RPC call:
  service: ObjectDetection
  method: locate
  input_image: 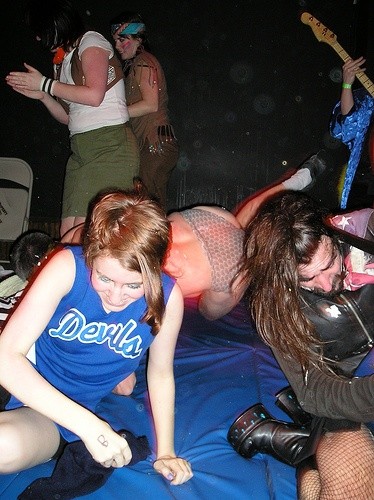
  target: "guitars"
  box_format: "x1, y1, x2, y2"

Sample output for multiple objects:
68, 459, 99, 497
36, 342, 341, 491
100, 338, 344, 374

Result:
301, 12, 374, 99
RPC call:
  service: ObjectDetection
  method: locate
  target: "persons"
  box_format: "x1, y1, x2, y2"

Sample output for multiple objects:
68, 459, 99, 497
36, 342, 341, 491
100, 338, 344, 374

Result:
228, 188, 374, 499
330, 57, 374, 215
5, 0, 142, 240
0, 192, 194, 485
9, 148, 330, 321
111, 12, 180, 210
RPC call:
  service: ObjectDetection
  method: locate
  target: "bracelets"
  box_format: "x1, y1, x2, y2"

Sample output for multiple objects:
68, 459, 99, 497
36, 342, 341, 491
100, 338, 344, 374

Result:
40, 76, 59, 96
342, 83, 351, 89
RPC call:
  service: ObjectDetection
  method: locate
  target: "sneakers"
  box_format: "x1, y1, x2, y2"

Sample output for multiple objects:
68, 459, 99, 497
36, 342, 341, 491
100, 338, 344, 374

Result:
299, 148, 333, 193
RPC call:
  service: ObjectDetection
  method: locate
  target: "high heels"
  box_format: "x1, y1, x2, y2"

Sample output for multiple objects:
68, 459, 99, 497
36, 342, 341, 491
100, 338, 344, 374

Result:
226, 383, 322, 479
